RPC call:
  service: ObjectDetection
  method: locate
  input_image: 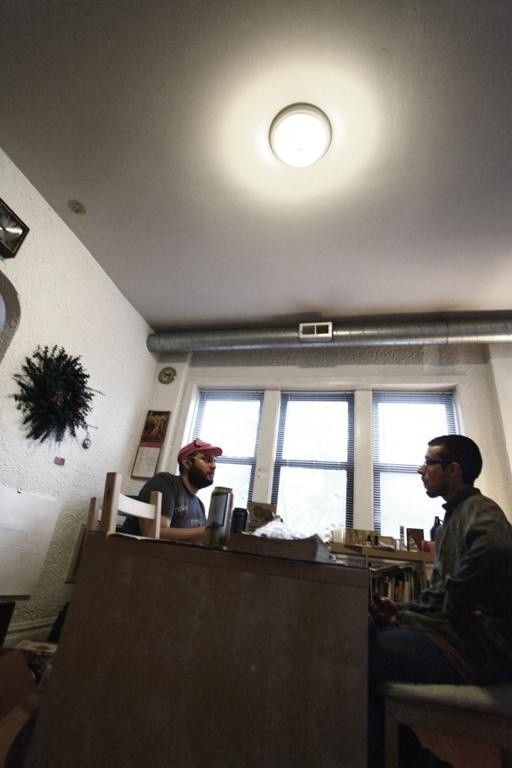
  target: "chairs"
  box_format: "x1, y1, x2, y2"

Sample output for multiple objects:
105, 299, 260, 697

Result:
83, 472, 163, 539
382, 679, 512, 767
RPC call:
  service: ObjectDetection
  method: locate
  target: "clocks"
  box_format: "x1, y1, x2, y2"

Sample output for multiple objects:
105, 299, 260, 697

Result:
0, 199, 30, 258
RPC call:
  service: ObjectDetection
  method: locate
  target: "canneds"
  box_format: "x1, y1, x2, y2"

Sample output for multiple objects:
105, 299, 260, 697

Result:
203, 486, 233, 543
231, 507, 248, 534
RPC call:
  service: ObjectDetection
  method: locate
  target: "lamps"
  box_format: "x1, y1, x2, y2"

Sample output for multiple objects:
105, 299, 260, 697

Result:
268, 102, 332, 169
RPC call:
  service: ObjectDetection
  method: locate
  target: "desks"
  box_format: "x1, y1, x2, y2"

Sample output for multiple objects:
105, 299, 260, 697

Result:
25, 535, 373, 767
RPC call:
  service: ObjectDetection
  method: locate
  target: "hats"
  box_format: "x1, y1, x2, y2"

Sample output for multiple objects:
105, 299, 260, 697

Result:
176, 437, 224, 465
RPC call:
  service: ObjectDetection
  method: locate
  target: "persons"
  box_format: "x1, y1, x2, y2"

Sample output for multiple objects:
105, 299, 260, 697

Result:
120, 439, 223, 544
372, 434, 511, 684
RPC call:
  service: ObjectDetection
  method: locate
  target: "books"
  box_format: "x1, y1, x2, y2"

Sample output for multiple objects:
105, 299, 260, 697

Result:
373, 569, 415, 602
